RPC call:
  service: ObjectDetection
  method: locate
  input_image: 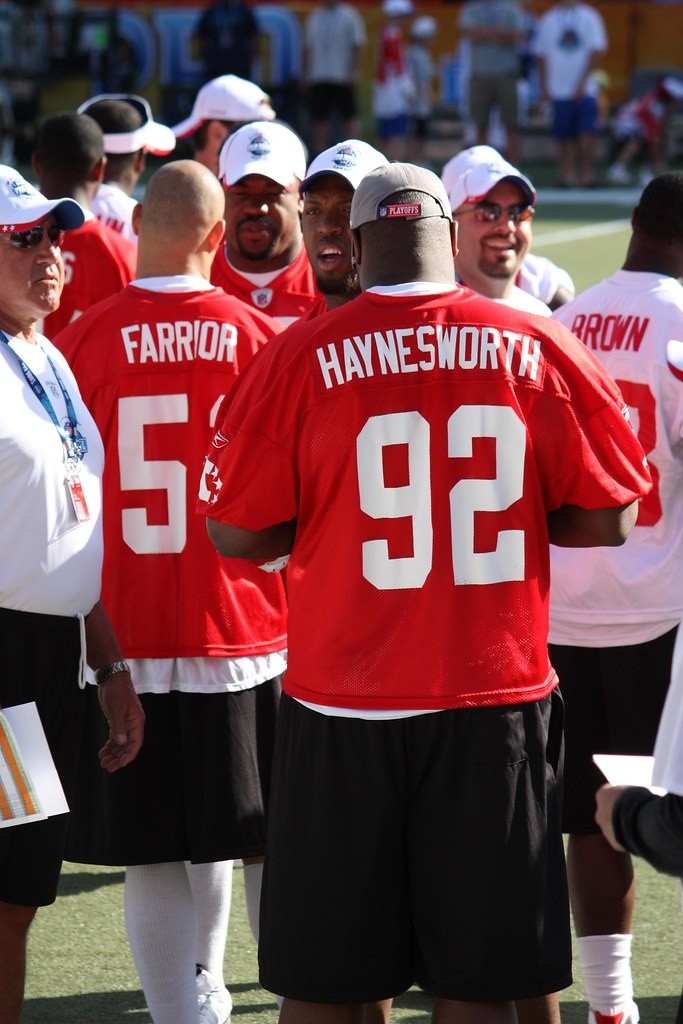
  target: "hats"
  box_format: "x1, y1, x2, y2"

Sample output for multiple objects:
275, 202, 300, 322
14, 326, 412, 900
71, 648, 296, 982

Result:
441, 147, 536, 213
170, 75, 275, 138
78, 94, 175, 154
296, 139, 389, 198
350, 161, 454, 227
0, 164, 85, 232
217, 120, 307, 191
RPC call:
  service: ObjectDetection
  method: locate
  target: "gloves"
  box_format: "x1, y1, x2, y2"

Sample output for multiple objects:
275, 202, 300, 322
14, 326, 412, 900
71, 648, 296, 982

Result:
558, 742, 603, 833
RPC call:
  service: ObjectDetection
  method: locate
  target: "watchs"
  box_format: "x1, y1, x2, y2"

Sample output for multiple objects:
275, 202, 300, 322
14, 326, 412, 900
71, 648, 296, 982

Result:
93, 661, 131, 683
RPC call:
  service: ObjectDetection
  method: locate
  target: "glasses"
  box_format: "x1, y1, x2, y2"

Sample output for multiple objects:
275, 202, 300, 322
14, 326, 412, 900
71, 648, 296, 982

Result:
0, 225, 65, 250
454, 202, 532, 224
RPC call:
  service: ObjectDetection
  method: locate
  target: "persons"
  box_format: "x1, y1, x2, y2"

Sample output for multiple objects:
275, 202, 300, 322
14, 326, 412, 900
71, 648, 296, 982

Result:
0, 163, 147, 1024
0, 1, 683, 188
31, 73, 391, 1024
0, 0, 121, 166
442, 144, 682, 1023
203, 159, 642, 1023
